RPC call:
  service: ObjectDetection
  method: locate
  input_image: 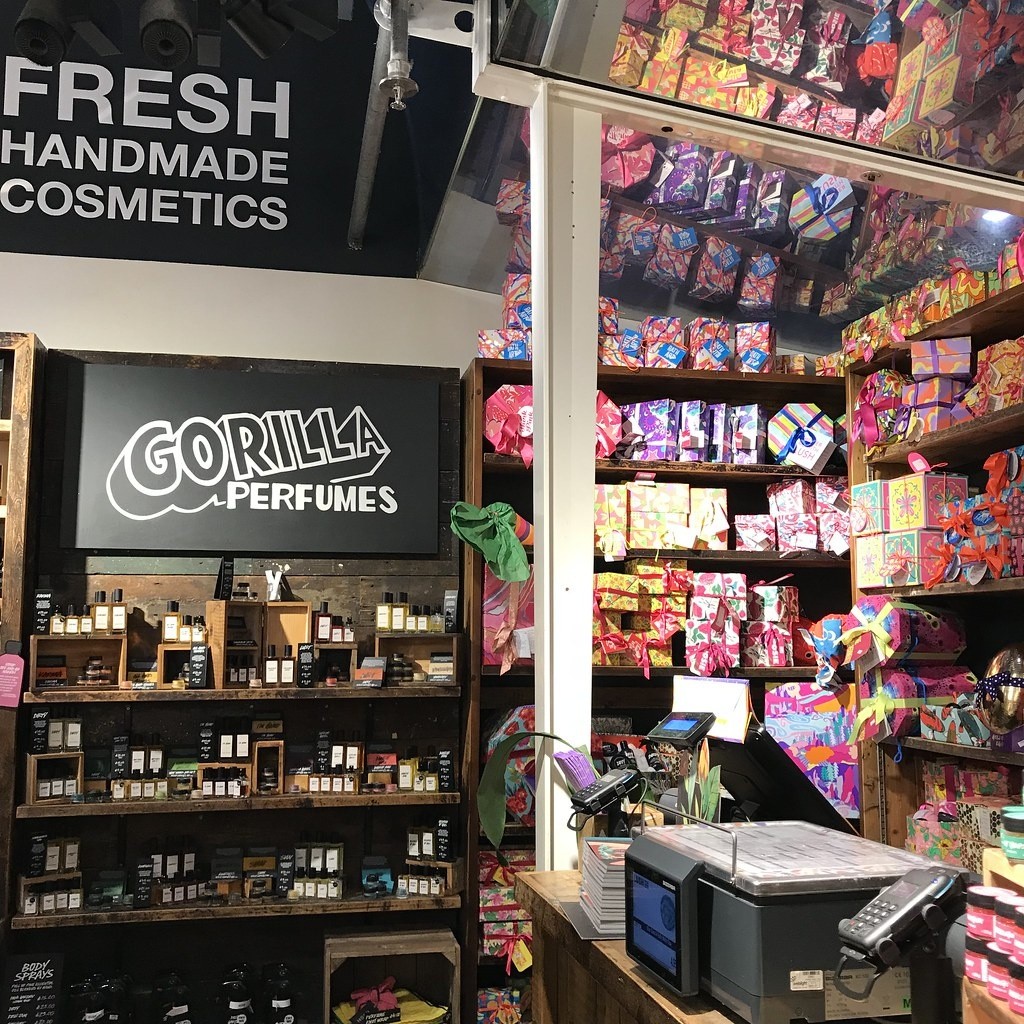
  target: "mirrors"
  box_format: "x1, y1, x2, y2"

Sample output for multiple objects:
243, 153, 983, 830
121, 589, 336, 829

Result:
417, 0, 1024, 357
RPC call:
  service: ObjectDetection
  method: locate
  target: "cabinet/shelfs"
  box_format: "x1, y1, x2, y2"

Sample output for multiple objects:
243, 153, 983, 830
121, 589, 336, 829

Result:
842, 284, 1024, 1024
9, 684, 468, 933
0, 331, 48, 943
461, 357, 853, 1024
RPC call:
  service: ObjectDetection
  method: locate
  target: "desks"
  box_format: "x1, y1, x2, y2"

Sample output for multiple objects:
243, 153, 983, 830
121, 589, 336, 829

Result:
510, 871, 982, 1024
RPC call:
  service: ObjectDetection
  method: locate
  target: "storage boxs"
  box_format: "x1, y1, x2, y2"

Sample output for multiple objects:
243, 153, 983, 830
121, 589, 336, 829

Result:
197, 761, 253, 797
29, 635, 128, 690
169, 778, 194, 793
323, 923, 461, 1024
367, 773, 392, 784
157, 644, 191, 689
266, 601, 313, 689
20, 752, 85, 804
85, 779, 106, 791
285, 775, 309, 792
376, 631, 467, 687
494, 0, 1024, 322
312, 645, 358, 686
204, 600, 263, 692
476, 235, 1024, 1024
251, 740, 284, 794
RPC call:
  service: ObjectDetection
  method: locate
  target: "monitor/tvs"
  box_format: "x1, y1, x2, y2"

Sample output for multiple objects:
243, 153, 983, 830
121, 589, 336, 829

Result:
623, 835, 704, 996
704, 723, 862, 839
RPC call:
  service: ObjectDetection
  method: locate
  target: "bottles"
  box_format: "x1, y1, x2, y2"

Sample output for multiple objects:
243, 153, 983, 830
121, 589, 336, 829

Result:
36, 706, 170, 806
31, 586, 210, 689
260, 767, 272, 797
171, 715, 254, 801
65, 958, 304, 1024
285, 827, 349, 902
221, 574, 360, 689
375, 589, 447, 686
287, 727, 441, 797
360, 814, 450, 902
20, 823, 280, 917
